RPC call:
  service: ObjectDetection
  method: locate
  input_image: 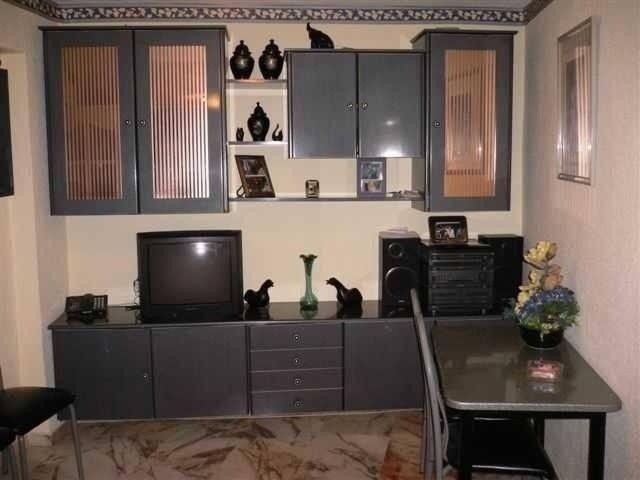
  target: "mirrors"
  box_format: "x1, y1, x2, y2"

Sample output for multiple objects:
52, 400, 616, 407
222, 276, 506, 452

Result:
558, 15, 595, 186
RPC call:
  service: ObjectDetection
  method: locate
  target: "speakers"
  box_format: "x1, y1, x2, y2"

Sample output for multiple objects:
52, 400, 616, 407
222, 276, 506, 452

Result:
378, 231, 421, 318
478, 234, 524, 316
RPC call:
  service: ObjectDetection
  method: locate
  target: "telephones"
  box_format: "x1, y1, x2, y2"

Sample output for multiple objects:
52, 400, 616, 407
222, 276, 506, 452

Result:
66, 294, 108, 323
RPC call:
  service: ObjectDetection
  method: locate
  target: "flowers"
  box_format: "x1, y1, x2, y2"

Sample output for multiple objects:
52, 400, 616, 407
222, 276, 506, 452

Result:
503, 240, 579, 340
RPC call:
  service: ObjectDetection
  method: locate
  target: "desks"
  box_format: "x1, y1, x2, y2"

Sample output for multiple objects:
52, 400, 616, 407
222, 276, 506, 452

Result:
430, 318, 622, 479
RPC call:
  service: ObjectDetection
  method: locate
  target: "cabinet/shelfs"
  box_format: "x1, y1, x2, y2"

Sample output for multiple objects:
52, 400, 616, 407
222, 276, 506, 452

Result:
283, 48, 426, 158
226, 77, 421, 203
344, 320, 441, 410
410, 30, 519, 212
38, 26, 230, 216
248, 325, 343, 416
47, 323, 248, 419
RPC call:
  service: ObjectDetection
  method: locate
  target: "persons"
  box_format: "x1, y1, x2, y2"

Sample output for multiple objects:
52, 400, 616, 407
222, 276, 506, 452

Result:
446, 225, 456, 240
362, 163, 383, 193
439, 225, 447, 239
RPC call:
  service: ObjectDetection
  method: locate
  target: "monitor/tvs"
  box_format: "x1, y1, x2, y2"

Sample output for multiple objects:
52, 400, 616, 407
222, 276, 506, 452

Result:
136, 230, 244, 323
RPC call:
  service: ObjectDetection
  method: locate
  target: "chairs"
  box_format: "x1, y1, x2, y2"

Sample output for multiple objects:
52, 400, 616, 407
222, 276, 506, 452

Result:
0, 369, 82, 480
409, 286, 558, 480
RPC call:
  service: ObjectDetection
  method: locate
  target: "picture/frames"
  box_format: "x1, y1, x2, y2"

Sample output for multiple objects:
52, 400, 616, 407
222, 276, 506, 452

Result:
357, 158, 386, 197
234, 155, 274, 198
428, 216, 468, 243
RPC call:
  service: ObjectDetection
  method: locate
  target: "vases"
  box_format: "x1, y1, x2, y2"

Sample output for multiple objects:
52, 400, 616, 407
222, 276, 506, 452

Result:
517, 325, 565, 350
298, 254, 319, 310
